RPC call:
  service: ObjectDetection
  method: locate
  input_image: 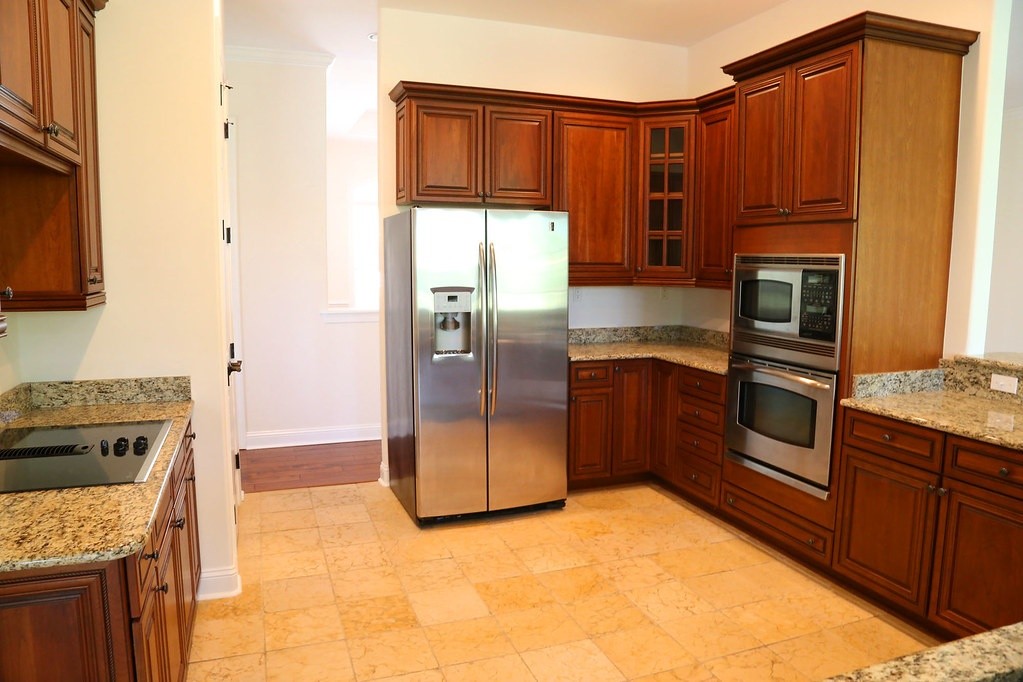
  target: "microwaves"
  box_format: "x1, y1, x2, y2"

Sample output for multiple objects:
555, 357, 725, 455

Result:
729, 252, 845, 373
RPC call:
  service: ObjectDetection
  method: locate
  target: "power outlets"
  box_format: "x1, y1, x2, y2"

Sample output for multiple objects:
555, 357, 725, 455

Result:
573, 287, 584, 302
990, 372, 1018, 395
659, 286, 672, 303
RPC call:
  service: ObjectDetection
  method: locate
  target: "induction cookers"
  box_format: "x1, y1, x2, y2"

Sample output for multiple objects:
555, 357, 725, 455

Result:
0, 417, 174, 492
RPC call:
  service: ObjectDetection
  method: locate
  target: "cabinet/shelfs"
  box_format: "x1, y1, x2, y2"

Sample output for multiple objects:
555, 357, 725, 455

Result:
171, 439, 198, 663
632, 98, 696, 288
552, 93, 635, 288
0, 477, 188, 682
0, 0, 82, 177
829, 407, 1023, 642
568, 361, 613, 492
183, 421, 201, 601
389, 79, 553, 207
694, 85, 733, 291
674, 365, 727, 516
612, 358, 652, 484
718, 457, 835, 577
0, 0, 109, 312
719, 10, 981, 228
649, 359, 678, 491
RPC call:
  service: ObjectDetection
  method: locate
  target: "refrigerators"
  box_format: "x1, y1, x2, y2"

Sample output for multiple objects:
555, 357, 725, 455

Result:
382, 206, 569, 528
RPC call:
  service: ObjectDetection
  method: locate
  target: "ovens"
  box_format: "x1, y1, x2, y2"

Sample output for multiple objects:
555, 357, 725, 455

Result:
723, 353, 837, 501
